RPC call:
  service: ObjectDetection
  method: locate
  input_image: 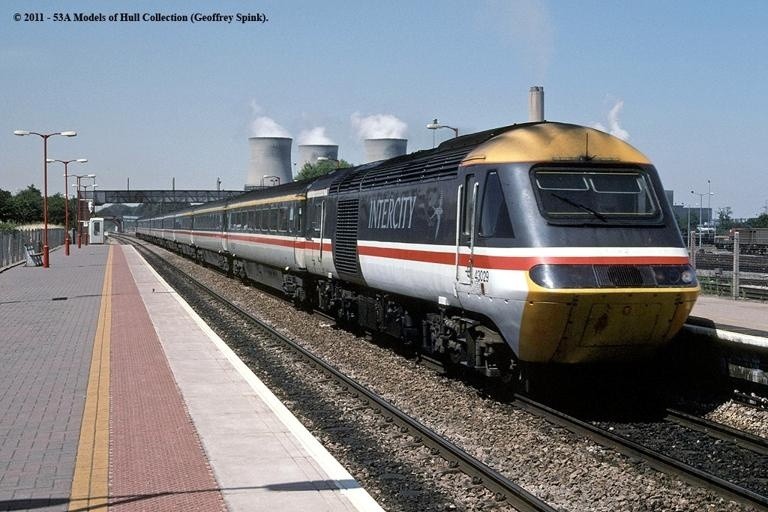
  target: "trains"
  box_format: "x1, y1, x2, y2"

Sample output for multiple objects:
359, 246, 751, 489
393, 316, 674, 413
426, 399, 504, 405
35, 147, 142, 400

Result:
134, 115, 705, 392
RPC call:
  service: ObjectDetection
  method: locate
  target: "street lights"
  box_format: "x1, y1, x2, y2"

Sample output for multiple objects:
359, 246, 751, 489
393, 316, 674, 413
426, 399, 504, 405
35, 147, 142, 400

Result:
63, 174, 98, 248
683, 181, 713, 246
45, 159, 88, 256
14, 129, 77, 268
426, 122, 462, 142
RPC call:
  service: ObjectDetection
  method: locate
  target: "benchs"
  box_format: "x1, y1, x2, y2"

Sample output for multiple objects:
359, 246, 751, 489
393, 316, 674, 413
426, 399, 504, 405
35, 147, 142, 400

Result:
23, 241, 44, 267
